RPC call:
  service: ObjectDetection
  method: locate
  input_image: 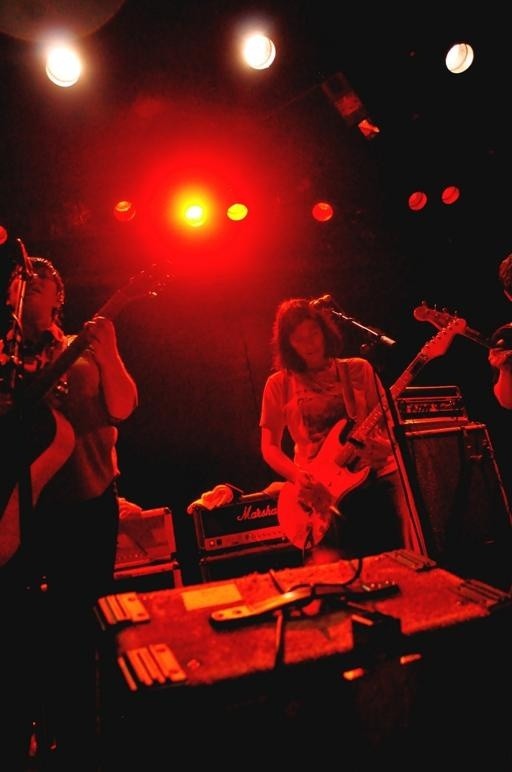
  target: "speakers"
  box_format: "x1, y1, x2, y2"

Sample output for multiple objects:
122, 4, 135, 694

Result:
404, 422, 512, 591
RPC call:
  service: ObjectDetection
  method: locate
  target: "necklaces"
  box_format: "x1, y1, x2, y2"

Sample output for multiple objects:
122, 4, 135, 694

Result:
305, 361, 330, 374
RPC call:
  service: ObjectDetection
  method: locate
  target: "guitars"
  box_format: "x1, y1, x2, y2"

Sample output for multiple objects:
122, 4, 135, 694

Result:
414, 300, 510, 353
4, 262, 175, 560
277, 318, 468, 550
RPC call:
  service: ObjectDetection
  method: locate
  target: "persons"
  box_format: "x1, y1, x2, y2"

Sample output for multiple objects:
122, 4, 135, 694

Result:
256, 291, 399, 563
484, 251, 512, 414
0, 251, 143, 769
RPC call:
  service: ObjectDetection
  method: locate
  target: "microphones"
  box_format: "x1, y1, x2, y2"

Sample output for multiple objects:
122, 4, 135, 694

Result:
16, 237, 39, 286
308, 295, 331, 312
